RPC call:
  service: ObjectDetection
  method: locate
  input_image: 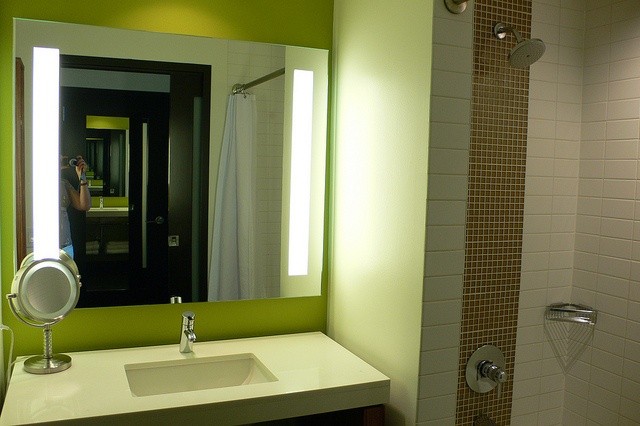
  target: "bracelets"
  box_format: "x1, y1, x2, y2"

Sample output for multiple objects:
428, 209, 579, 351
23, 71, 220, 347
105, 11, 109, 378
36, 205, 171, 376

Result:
79, 182, 87, 186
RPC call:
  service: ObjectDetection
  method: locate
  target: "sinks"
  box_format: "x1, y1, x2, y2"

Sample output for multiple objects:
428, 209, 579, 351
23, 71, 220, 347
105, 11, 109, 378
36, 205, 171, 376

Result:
124, 351, 280, 399
90, 208, 119, 210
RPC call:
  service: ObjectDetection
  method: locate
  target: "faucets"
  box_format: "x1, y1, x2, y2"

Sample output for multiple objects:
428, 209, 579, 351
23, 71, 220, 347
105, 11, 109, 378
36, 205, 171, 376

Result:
184, 329, 196, 342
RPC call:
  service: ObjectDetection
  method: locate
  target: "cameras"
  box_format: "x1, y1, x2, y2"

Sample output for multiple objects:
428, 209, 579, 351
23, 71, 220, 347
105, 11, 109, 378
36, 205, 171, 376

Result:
63, 157, 79, 167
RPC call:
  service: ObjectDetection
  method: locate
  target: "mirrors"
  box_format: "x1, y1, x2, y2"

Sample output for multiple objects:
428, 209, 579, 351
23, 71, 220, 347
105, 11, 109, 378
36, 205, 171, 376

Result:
19, 250, 79, 278
7, 258, 82, 375
12, 17, 329, 308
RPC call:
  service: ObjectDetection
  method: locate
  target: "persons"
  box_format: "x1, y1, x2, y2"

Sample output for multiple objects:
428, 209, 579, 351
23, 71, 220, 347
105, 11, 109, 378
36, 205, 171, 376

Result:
60, 154, 91, 260
